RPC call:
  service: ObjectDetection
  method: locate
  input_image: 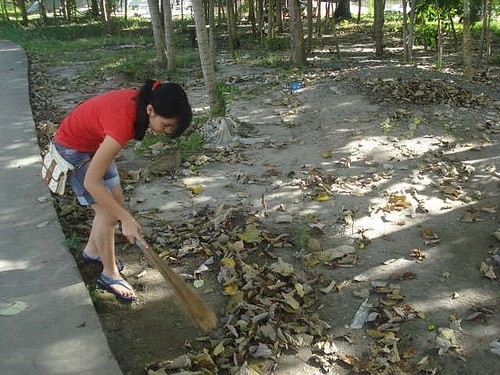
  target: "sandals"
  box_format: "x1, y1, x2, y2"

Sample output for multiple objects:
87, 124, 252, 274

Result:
81, 249, 124, 272
97, 272, 137, 302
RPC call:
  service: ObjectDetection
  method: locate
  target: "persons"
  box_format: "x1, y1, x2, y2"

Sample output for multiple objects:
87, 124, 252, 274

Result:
41, 79, 194, 301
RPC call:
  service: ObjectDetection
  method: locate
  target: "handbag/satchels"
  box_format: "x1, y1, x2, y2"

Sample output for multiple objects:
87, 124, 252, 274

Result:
41, 139, 76, 196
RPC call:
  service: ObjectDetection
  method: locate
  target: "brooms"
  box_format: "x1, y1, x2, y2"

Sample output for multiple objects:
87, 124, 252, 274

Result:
136, 236, 217, 332
